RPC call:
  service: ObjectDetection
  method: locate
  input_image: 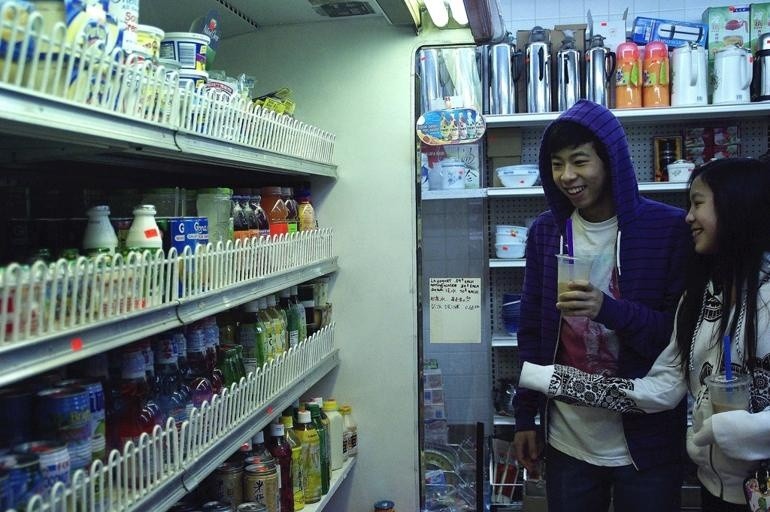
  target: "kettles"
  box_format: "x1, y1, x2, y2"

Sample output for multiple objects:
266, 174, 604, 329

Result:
671, 33, 770, 106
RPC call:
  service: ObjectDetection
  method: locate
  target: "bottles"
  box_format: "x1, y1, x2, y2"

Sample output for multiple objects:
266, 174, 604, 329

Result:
490, 25, 670, 111
82, 186, 358, 512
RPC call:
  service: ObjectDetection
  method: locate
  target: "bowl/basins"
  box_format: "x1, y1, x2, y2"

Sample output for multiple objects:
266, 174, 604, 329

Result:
500, 292, 519, 338
494, 224, 527, 259
667, 159, 696, 184
494, 163, 540, 188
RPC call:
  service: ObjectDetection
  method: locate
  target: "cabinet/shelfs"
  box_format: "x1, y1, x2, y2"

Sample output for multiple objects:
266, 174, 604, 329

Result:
484, 100, 770, 510
0, 77, 357, 512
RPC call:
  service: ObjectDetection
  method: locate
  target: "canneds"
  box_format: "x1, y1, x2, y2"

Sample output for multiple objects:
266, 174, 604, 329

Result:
6, 215, 208, 299
0, 375, 109, 512
169, 456, 281, 512
374, 500, 397, 512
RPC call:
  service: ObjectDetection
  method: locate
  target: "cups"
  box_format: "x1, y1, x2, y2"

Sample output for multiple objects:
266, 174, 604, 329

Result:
557, 252, 593, 313
656, 21, 703, 44
703, 374, 753, 413
440, 157, 465, 188
659, 137, 676, 179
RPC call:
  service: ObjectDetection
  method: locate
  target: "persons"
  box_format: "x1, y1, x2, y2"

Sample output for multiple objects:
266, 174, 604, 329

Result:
512, 99, 696, 512
513, 155, 770, 512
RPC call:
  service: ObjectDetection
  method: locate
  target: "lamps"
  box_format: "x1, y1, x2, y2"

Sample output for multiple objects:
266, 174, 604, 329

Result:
421, 0, 470, 29
